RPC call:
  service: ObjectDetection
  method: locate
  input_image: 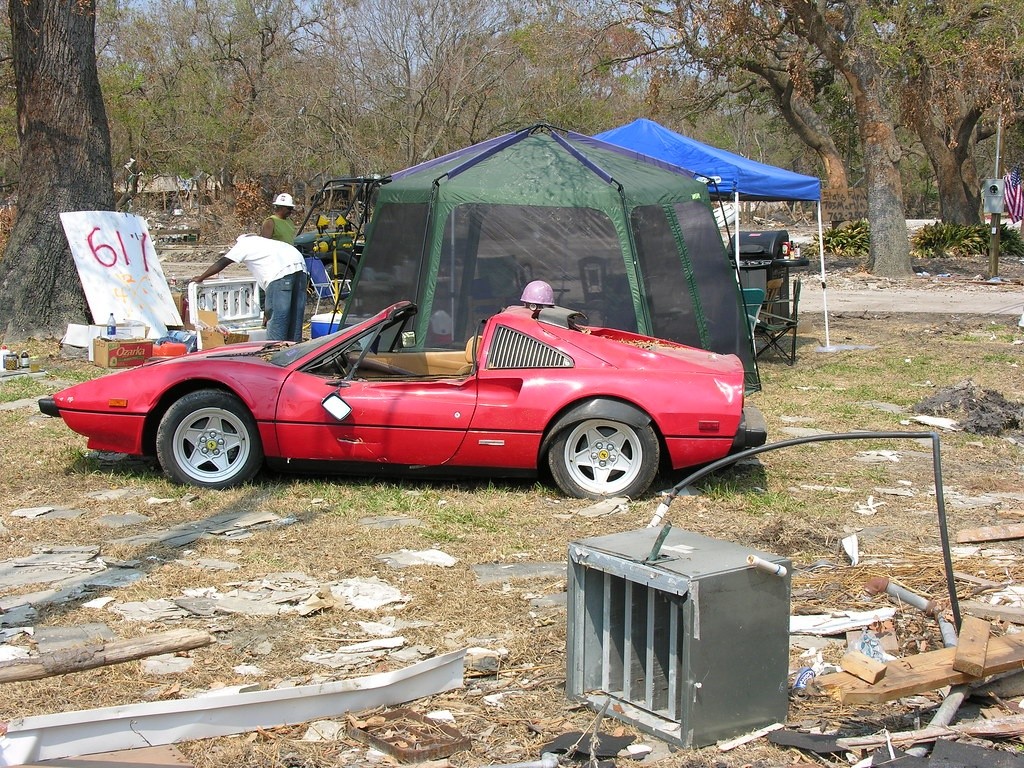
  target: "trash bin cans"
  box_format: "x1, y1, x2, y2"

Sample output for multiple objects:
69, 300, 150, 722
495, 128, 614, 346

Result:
724, 230, 790, 324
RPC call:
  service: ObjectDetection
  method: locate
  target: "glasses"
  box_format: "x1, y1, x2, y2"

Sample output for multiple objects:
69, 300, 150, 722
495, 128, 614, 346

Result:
282, 206, 293, 210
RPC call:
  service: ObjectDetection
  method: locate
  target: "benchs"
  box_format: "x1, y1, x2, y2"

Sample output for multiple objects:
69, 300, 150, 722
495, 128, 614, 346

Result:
148, 229, 201, 245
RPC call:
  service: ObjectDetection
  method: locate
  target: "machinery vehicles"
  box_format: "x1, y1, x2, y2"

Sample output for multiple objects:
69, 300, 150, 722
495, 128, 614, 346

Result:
295, 176, 394, 300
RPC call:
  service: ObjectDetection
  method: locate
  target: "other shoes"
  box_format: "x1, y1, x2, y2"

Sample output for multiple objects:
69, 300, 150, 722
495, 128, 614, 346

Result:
262, 322, 267, 328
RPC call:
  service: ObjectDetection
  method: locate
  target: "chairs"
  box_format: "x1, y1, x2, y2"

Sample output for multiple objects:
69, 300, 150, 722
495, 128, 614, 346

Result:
758, 278, 783, 350
304, 255, 352, 315
465, 297, 507, 346
576, 256, 608, 328
456, 335, 484, 376
741, 288, 766, 370
755, 280, 803, 366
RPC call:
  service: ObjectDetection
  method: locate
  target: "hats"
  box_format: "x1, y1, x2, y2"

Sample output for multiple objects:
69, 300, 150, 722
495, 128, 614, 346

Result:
235, 233, 257, 244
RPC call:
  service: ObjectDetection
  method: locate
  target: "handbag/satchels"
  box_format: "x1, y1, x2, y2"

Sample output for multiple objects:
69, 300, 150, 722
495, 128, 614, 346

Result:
155, 330, 197, 354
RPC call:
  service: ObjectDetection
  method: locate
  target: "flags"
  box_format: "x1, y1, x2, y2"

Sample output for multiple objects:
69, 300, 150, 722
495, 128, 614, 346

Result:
1002, 167, 1024, 222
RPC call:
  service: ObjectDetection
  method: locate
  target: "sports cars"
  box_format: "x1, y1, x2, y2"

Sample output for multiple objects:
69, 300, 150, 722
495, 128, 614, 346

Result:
37, 300, 768, 495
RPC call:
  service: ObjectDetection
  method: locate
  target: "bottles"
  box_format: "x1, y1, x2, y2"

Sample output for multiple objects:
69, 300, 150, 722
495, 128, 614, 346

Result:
789, 241, 795, 260
782, 241, 790, 259
0, 345, 29, 370
107, 313, 117, 341
794, 242, 800, 260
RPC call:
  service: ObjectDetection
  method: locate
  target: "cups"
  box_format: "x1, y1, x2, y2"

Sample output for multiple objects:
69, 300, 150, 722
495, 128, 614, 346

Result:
30, 360, 39, 372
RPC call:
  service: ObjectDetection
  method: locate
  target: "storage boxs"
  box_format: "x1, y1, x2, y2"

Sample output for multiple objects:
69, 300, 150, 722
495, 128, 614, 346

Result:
197, 311, 250, 350
188, 276, 274, 349
93, 336, 153, 368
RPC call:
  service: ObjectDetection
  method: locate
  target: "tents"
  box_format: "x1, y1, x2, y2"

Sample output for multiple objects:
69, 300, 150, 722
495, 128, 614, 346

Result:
327, 117, 829, 389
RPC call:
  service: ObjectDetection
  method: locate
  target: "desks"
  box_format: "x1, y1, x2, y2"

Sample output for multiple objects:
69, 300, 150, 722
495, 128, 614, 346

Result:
730, 258, 773, 288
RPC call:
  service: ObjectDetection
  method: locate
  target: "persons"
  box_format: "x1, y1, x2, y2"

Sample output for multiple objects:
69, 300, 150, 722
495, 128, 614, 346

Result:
259, 193, 295, 330
191, 231, 308, 341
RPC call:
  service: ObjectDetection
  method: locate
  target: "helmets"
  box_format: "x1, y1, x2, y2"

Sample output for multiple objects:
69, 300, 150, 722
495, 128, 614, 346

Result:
520, 280, 554, 305
272, 193, 295, 206
